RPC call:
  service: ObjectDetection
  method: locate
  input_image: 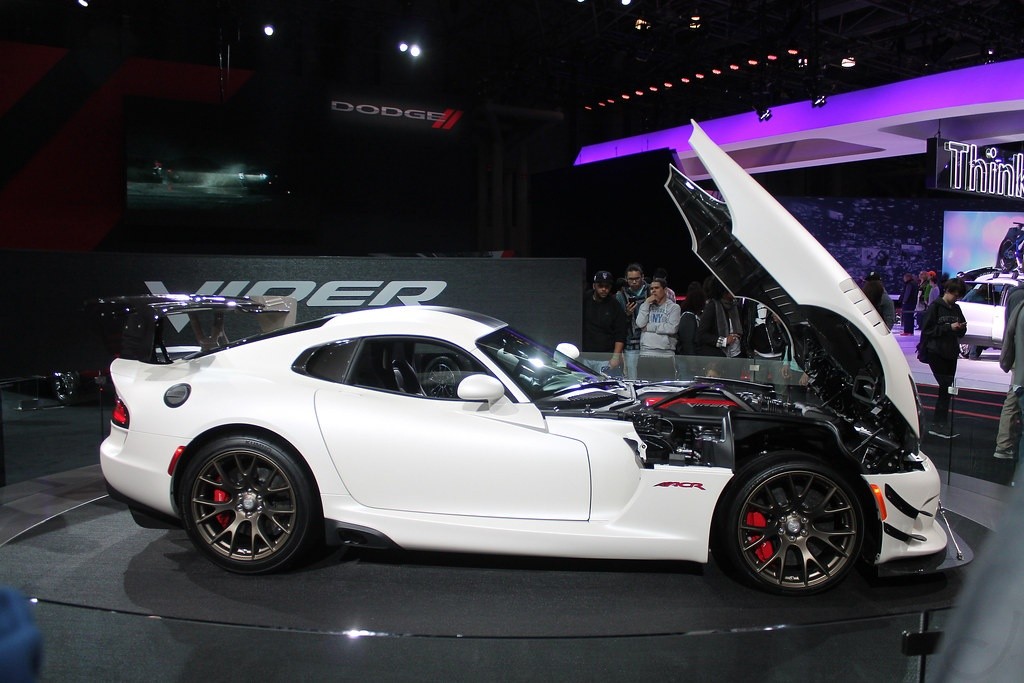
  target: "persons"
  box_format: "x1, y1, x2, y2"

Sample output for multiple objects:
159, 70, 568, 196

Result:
900, 271, 940, 336
616, 265, 651, 380
679, 280, 705, 379
704, 363, 722, 378
699, 277, 746, 358
782, 345, 817, 401
653, 269, 675, 303
957, 272, 964, 278
916, 278, 968, 438
676, 282, 706, 381
999, 300, 1023, 487
582, 272, 626, 377
993, 284, 1024, 459
740, 299, 789, 395
864, 272, 895, 330
635, 278, 681, 381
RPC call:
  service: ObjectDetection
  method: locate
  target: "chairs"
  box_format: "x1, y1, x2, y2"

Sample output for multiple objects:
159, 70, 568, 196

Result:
392, 358, 427, 397
374, 369, 407, 393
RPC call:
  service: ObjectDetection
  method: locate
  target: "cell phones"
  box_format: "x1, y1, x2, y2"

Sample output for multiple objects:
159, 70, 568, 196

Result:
628, 296, 636, 307
962, 322, 967, 325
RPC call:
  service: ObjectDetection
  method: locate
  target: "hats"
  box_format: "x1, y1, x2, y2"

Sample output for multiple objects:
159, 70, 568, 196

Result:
926, 271, 936, 277
864, 272, 881, 282
593, 271, 613, 283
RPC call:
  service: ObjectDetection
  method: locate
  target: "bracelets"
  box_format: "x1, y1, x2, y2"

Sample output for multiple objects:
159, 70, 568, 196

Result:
612, 356, 619, 359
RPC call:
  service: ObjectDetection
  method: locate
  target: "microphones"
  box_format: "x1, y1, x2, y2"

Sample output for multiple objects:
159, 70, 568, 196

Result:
617, 278, 628, 287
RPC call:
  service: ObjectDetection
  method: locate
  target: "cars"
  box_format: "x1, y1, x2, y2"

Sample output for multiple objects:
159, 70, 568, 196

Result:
955, 270, 1023, 361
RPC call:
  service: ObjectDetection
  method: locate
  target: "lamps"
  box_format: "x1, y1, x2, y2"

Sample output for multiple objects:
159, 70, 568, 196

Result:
809, 93, 827, 108
751, 105, 772, 122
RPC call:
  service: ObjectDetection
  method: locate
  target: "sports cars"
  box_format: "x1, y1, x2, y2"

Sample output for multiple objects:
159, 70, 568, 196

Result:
98, 114, 977, 595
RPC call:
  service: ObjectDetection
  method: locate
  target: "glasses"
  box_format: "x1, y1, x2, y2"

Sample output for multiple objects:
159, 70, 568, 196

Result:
627, 277, 642, 283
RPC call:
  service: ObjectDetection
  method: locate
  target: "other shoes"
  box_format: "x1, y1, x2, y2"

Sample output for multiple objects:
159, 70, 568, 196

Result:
927, 423, 960, 439
900, 332, 913, 336
915, 327, 921, 330
993, 448, 1013, 458
